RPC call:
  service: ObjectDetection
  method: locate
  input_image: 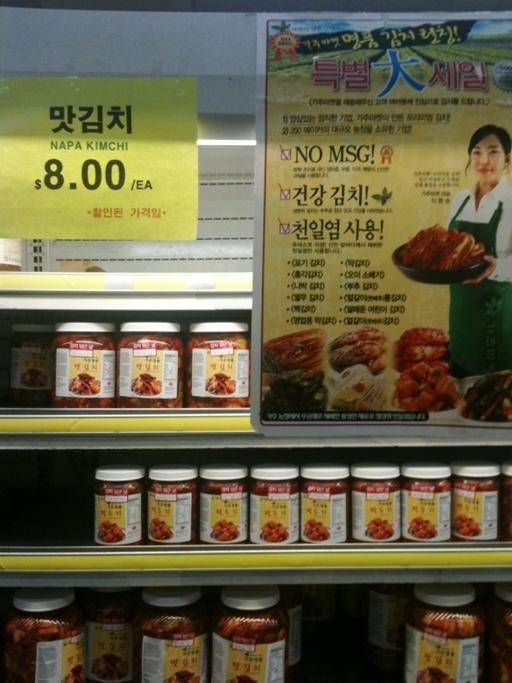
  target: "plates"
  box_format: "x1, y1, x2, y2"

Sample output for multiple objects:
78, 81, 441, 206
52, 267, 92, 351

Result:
261, 336, 507, 427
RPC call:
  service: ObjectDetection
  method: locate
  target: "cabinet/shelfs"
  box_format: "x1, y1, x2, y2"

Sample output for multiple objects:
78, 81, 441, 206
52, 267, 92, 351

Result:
0, 272, 512, 682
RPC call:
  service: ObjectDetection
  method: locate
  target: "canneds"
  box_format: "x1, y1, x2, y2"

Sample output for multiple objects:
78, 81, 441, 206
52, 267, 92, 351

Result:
451, 461, 501, 541
115, 321, 186, 408
501, 461, 512, 540
93, 463, 145, 546
400, 460, 451, 541
50, 322, 115, 408
147, 464, 199, 545
6, 323, 54, 407
249, 463, 299, 543
350, 461, 400, 542
199, 462, 248, 544
185, 322, 250, 408
299, 460, 350, 543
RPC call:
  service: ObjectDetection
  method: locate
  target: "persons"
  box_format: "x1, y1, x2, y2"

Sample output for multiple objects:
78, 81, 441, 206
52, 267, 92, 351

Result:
446, 124, 511, 378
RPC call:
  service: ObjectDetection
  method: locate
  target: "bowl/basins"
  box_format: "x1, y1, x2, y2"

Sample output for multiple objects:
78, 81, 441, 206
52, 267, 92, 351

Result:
392, 241, 487, 284
